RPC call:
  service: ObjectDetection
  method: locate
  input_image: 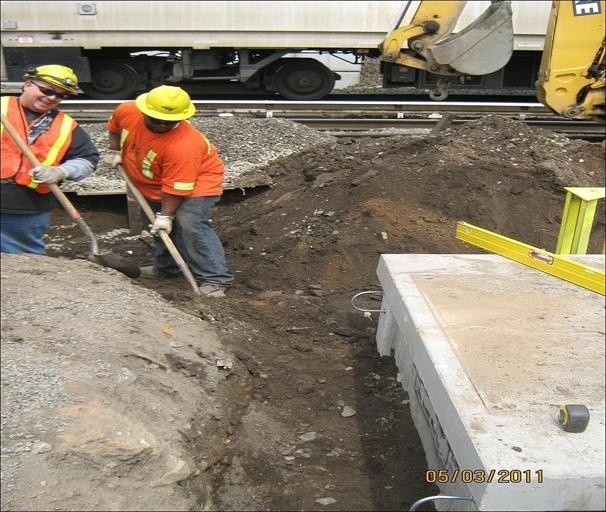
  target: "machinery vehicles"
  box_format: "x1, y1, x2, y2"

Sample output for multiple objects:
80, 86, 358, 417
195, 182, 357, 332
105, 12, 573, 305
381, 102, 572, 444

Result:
377, 0, 606, 119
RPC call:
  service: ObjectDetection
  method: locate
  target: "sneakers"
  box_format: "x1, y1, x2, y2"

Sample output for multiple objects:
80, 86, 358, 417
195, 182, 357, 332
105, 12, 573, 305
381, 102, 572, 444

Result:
137, 264, 181, 281
198, 282, 227, 300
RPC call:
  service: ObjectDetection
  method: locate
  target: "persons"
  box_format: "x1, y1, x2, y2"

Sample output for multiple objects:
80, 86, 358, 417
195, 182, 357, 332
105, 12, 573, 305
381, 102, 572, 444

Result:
105, 85, 235, 297
0, 65, 100, 254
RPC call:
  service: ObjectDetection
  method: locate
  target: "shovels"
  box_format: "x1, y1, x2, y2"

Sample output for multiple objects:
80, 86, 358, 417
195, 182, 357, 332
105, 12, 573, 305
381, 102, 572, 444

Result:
104, 153, 226, 298
1, 112, 142, 278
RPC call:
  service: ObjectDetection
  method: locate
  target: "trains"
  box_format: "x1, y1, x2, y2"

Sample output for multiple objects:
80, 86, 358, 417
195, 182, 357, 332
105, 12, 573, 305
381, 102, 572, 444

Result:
0, 0, 553, 101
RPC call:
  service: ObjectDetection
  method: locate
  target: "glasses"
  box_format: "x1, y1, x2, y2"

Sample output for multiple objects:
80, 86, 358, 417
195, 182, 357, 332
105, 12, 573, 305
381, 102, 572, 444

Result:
29, 80, 71, 100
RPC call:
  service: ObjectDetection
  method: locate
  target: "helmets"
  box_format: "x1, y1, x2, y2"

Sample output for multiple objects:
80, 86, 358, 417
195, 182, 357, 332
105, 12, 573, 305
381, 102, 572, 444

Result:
135, 84, 196, 121
23, 64, 83, 96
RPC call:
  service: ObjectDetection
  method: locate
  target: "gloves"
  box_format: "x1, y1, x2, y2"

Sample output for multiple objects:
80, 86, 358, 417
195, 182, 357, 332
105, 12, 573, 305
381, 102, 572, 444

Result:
102, 147, 124, 170
28, 164, 67, 184
148, 211, 176, 238
